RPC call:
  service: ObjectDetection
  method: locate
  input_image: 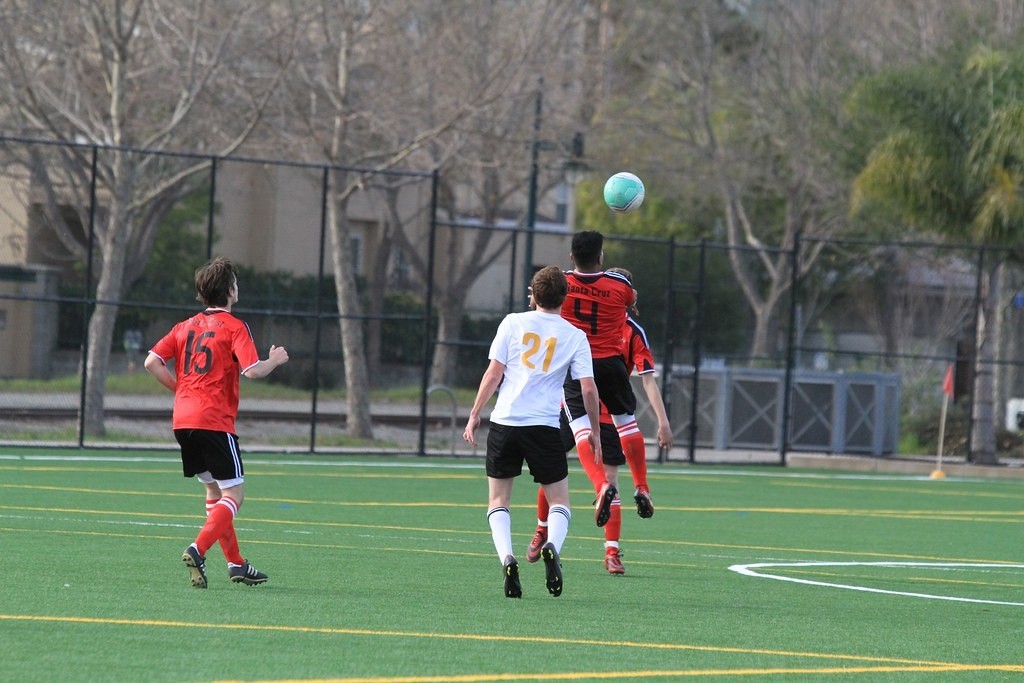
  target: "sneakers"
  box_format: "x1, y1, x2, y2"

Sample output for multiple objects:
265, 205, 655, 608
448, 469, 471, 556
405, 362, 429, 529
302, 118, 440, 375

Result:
182, 546, 207, 589
541, 542, 563, 597
604, 546, 625, 575
503, 554, 522, 599
526, 524, 548, 563
227, 559, 269, 586
591, 482, 618, 527
634, 486, 653, 519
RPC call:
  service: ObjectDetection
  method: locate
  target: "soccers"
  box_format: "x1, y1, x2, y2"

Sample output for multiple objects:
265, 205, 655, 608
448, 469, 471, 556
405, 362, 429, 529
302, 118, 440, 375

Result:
604, 171, 645, 213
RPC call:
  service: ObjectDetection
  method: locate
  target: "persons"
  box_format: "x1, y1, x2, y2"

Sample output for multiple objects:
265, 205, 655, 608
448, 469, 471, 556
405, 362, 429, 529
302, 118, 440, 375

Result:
144, 257, 289, 589
462, 266, 601, 598
526, 230, 673, 574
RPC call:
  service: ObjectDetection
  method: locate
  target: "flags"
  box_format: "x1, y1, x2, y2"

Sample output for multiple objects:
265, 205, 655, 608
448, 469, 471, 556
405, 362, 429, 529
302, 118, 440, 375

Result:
941, 364, 955, 398
